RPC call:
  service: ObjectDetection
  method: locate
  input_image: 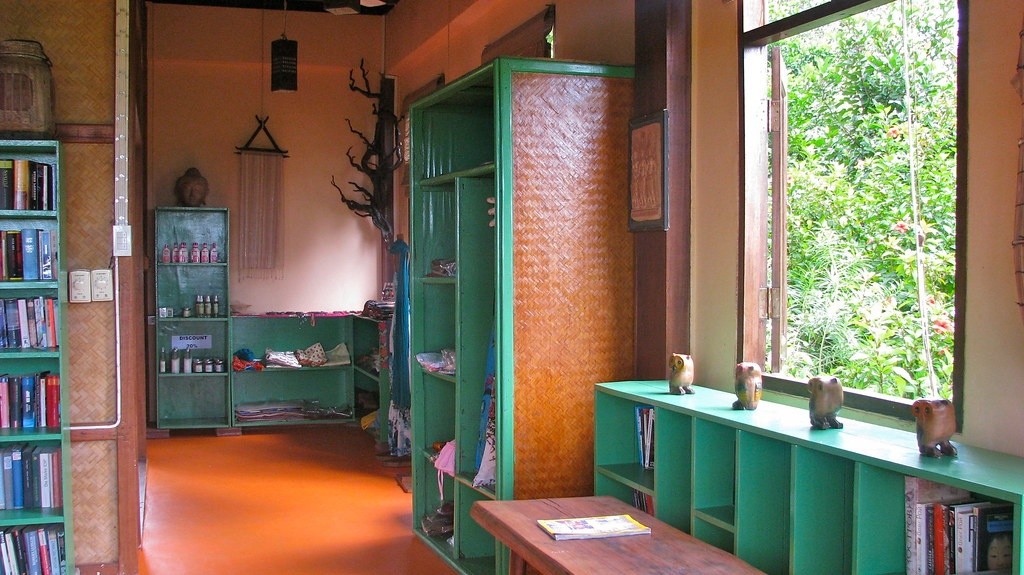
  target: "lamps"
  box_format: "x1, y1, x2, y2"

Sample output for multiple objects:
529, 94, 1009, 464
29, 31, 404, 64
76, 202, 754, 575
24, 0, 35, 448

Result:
270, 0, 299, 95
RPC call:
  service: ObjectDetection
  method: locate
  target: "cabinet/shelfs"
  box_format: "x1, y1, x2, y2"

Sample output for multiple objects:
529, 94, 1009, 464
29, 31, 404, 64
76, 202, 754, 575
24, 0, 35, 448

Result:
154, 205, 232, 429
229, 312, 357, 426
0, 138, 76, 575
593, 379, 1024, 575
351, 311, 390, 443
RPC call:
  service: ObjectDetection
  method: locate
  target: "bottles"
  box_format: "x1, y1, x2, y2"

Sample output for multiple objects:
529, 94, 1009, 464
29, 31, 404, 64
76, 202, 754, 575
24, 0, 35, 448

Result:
171, 348, 180, 373
183, 348, 192, 373
160, 347, 166, 373
196, 295, 218, 318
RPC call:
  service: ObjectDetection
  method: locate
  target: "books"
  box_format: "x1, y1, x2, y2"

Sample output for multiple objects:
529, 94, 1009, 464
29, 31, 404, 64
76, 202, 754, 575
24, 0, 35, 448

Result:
536, 514, 652, 541
633, 489, 654, 516
0, 158, 67, 575
905, 475, 1014, 575
634, 405, 655, 469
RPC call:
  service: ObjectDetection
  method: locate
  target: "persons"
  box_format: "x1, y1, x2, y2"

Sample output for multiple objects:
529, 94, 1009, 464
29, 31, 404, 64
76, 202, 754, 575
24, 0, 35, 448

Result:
174, 168, 209, 207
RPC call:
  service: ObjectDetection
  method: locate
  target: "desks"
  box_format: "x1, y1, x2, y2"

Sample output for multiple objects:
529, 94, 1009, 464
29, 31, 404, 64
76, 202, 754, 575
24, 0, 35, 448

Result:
408, 56, 640, 575
468, 493, 772, 575
